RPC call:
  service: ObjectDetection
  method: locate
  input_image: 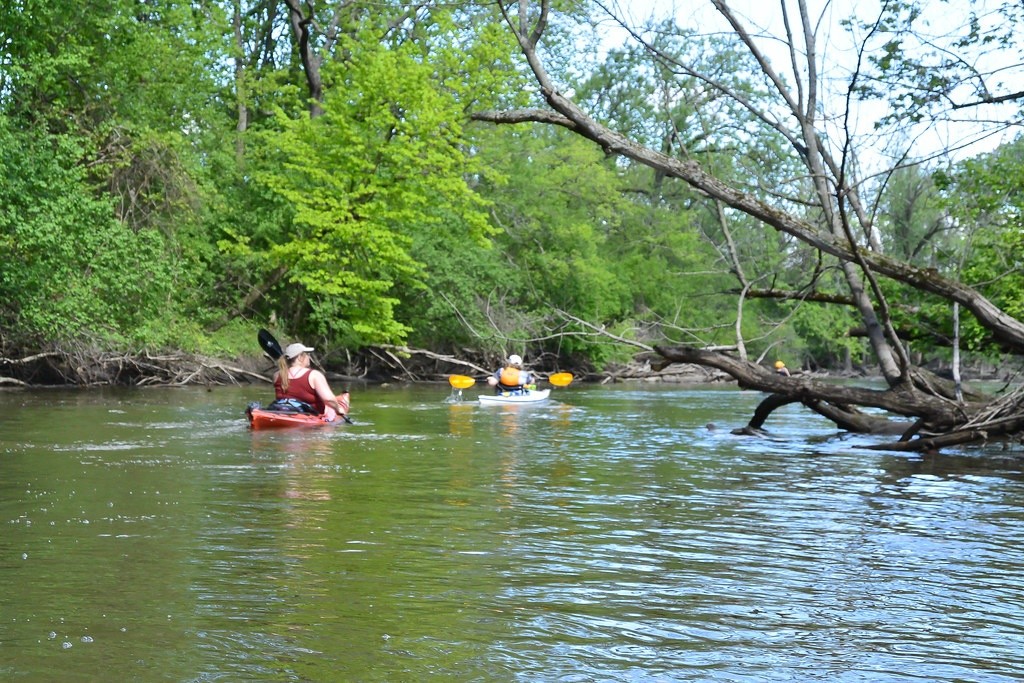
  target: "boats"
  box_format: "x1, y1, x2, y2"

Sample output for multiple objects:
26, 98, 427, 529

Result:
478, 387, 552, 404
246, 390, 356, 432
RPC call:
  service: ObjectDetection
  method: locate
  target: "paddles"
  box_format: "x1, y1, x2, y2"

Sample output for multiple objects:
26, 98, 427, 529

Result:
447, 372, 574, 390
257, 326, 355, 425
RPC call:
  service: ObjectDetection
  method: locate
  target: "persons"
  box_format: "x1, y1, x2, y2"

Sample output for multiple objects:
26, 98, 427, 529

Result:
273, 343, 346, 416
774, 361, 790, 376
486, 354, 535, 396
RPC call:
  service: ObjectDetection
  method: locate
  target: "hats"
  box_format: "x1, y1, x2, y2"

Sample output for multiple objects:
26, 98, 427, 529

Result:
509, 355, 522, 367
285, 343, 315, 359
